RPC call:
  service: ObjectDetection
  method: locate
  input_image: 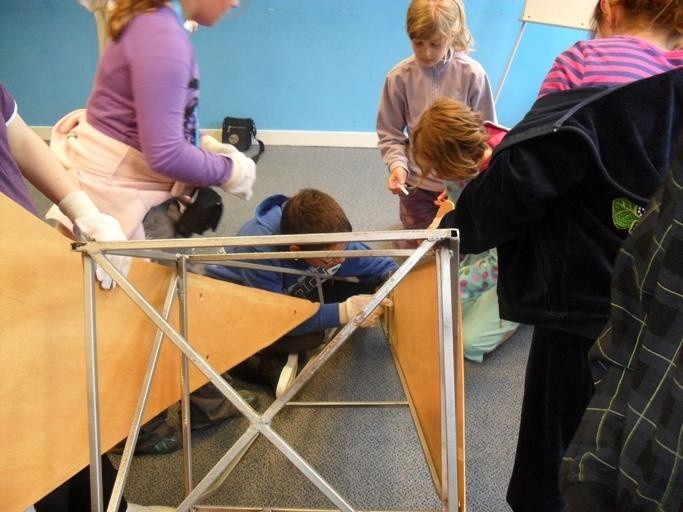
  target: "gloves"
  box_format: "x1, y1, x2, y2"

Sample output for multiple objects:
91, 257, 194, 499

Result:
339, 294, 395, 329
217, 153, 259, 201
199, 135, 239, 158
57, 191, 131, 290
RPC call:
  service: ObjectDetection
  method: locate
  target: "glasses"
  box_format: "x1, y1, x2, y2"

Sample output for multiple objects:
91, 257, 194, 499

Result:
318, 243, 349, 264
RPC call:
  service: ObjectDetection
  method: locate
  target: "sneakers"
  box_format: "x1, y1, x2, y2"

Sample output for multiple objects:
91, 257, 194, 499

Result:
259, 351, 300, 398
190, 388, 261, 431
125, 500, 177, 512
110, 434, 184, 458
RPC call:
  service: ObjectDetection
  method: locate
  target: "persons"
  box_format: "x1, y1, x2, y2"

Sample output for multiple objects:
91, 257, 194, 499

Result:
0, 80, 185, 511
399, 0, 682, 512
375, 0, 502, 254
193, 183, 403, 400
404, 96, 528, 366
56, 1, 261, 457
